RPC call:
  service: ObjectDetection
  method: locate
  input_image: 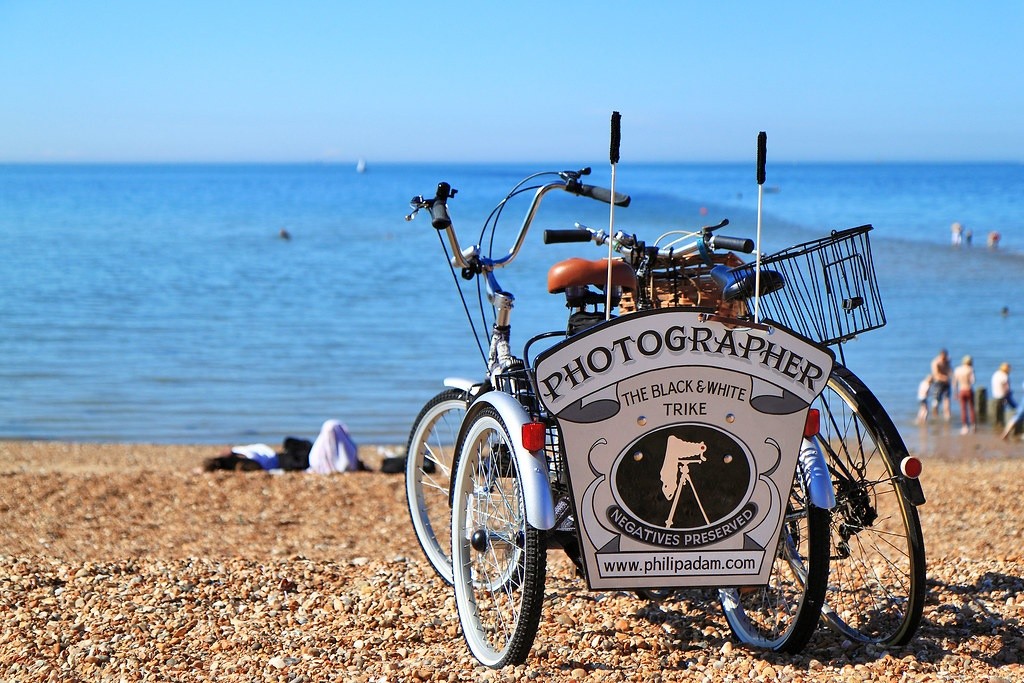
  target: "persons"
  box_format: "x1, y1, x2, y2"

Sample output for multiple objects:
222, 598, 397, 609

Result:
916, 374, 933, 425
965, 229, 973, 242
954, 355, 976, 434
930, 350, 953, 422
990, 231, 1001, 247
992, 363, 1011, 427
952, 223, 963, 245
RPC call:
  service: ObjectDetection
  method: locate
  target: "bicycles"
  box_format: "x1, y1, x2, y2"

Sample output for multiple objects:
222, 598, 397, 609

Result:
538, 214, 928, 652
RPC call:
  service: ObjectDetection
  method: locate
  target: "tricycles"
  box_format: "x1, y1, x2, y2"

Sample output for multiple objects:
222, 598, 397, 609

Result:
401, 167, 839, 670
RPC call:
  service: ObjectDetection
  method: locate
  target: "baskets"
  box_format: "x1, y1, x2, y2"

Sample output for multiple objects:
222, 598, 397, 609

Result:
725, 223, 886, 349
